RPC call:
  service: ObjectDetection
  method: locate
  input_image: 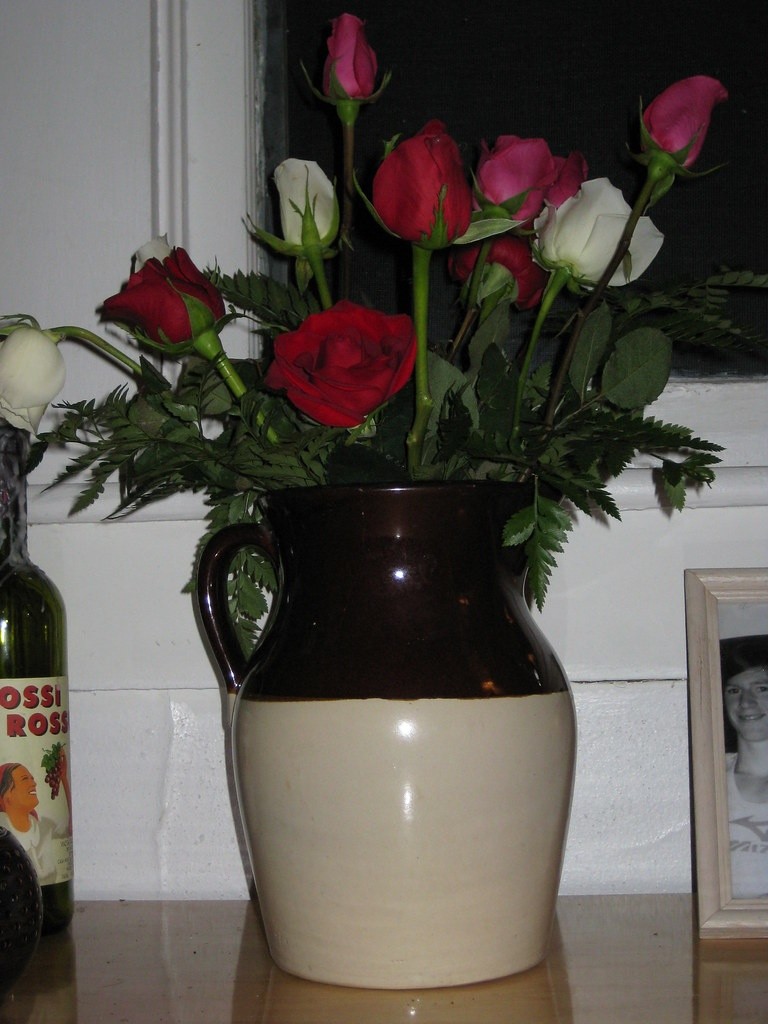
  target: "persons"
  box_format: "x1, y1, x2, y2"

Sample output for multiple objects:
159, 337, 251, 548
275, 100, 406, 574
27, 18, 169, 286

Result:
719, 634, 768, 899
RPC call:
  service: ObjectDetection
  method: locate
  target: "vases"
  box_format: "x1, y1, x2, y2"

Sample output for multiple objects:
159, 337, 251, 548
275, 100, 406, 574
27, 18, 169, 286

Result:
196, 479, 575, 990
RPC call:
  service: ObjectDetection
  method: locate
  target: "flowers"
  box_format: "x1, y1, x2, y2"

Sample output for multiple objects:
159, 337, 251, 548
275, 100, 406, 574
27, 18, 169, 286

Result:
0, 8, 766, 666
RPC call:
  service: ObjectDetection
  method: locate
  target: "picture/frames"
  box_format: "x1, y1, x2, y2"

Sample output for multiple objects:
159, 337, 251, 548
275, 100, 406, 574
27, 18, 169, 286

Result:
692, 938, 768, 1024
683, 567, 768, 939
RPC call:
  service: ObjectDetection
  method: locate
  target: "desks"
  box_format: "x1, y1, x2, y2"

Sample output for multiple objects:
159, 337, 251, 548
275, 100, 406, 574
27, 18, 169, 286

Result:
0, 898, 768, 1024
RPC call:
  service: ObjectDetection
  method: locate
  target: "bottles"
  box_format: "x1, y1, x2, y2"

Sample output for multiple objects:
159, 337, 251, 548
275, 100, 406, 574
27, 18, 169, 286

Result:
0, 418, 74, 935
